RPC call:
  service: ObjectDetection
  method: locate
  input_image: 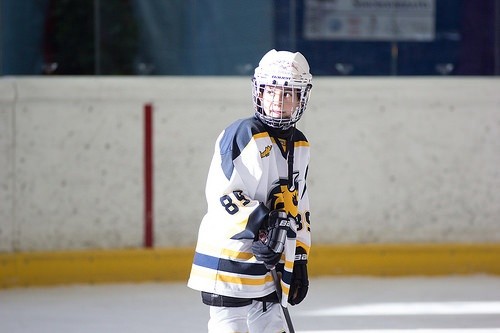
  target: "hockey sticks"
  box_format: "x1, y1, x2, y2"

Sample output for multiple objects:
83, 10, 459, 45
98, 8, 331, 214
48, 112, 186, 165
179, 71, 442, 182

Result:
259, 231, 297, 333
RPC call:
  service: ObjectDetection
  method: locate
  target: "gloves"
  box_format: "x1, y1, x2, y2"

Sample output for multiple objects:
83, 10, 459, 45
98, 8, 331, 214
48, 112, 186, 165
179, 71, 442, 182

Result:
251, 211, 290, 265
287, 247, 309, 306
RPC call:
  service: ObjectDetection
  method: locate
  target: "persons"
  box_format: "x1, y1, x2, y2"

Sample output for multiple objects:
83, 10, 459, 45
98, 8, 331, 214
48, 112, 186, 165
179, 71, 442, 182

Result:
185, 48, 313, 332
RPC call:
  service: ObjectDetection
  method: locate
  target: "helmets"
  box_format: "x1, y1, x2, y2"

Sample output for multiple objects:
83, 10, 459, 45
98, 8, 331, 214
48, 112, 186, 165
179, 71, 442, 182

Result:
252, 49, 313, 129
